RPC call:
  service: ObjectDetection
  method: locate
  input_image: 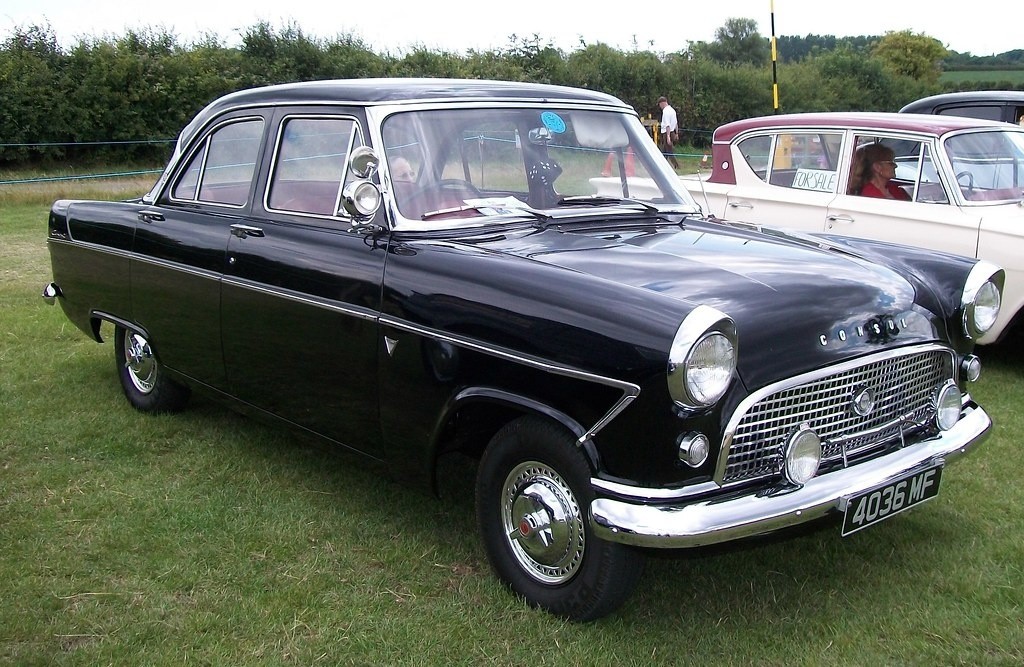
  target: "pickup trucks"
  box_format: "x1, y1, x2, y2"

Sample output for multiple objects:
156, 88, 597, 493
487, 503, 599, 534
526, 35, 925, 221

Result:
590, 110, 1023, 360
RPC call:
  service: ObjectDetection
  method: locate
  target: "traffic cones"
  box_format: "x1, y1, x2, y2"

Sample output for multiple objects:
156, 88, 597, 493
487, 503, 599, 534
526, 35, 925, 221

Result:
698, 153, 708, 169
625, 147, 635, 178
600, 154, 613, 177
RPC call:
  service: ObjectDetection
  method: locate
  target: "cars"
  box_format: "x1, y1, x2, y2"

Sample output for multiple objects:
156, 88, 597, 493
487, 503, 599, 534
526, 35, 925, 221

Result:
821, 91, 1023, 190
41, 78, 993, 625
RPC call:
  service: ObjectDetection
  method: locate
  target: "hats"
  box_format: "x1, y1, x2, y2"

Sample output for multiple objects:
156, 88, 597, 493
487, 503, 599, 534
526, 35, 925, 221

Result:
656, 97, 667, 105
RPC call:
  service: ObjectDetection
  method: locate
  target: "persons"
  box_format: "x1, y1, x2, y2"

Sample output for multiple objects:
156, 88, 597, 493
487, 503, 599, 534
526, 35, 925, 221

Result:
861, 147, 910, 201
389, 156, 415, 182
656, 97, 679, 168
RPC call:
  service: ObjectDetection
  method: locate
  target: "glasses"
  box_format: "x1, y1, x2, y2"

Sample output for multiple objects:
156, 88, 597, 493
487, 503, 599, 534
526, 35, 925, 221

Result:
396, 171, 415, 179
879, 160, 895, 167
